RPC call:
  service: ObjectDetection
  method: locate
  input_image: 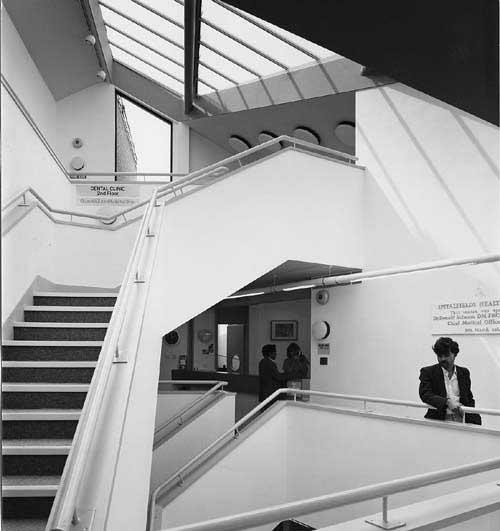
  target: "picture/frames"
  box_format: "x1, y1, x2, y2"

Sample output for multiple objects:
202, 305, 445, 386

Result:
271, 320, 298, 341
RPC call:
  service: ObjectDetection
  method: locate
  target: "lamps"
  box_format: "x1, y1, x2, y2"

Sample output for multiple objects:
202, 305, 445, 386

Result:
84, 35, 106, 82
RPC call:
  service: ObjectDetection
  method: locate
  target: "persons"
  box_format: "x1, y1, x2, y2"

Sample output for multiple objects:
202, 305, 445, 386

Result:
419, 335, 482, 426
258, 343, 287, 412
283, 341, 309, 379
